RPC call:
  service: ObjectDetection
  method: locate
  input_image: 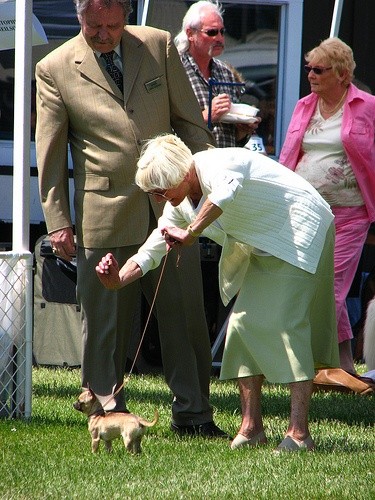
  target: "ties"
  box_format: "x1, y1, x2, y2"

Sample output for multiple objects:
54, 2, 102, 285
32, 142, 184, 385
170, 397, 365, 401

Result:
101, 50, 124, 95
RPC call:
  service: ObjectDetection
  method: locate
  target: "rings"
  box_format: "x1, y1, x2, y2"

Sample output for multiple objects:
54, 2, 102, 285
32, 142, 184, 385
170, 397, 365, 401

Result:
52, 248, 56, 250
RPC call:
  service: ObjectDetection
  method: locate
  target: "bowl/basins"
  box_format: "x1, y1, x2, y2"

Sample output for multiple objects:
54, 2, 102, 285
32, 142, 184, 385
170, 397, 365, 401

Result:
230, 103, 260, 117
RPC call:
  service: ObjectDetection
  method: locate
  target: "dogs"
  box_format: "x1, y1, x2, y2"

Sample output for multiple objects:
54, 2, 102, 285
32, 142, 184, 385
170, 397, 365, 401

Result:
73, 382, 158, 455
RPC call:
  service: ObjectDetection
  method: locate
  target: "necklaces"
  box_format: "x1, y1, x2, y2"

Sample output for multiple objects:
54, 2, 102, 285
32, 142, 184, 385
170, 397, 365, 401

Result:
320, 87, 349, 113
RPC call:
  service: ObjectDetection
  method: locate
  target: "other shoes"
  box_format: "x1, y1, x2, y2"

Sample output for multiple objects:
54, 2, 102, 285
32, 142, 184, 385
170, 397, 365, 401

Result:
230, 433, 268, 451
273, 433, 315, 454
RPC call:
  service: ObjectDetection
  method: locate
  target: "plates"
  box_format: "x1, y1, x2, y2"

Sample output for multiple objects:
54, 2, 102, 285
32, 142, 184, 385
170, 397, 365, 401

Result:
222, 113, 259, 124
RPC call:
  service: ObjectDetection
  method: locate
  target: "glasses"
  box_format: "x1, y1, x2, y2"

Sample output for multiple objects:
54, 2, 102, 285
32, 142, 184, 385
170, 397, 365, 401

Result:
304, 65, 332, 74
147, 189, 166, 196
201, 28, 225, 36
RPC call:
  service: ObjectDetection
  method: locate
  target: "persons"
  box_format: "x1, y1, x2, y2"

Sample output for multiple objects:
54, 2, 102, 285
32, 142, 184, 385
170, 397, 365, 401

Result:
277, 37, 375, 395
35, 0, 234, 443
94, 134, 340, 451
174, 0, 262, 377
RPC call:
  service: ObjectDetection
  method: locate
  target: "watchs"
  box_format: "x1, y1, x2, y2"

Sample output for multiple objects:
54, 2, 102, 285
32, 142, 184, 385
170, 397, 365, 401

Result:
187, 223, 202, 237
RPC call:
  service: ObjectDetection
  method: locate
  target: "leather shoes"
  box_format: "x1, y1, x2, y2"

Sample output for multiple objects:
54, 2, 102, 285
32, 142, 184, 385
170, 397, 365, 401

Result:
172, 422, 234, 440
